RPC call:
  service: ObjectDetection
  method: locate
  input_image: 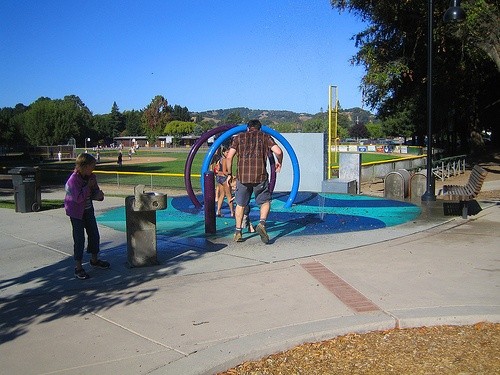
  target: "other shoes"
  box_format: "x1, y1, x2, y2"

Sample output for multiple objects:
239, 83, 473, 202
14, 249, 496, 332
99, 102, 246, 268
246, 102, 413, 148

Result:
216, 212, 225, 217
230, 212, 235, 218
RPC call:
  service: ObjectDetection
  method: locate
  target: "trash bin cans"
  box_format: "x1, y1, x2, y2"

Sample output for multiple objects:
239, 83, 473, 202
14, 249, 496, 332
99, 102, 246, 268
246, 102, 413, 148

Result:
8, 166, 42, 213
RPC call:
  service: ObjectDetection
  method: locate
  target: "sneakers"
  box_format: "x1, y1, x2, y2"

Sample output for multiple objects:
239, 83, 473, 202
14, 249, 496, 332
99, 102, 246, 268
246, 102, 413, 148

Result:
73, 268, 89, 280
89, 259, 110, 270
233, 233, 243, 242
248, 225, 255, 233
256, 223, 269, 244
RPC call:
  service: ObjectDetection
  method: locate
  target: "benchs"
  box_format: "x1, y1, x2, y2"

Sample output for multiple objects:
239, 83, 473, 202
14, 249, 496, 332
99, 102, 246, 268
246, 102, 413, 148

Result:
443, 165, 488, 220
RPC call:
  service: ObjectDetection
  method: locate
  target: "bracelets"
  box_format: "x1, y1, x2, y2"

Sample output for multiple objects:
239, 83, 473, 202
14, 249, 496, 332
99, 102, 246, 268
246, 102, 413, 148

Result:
228, 173, 232, 175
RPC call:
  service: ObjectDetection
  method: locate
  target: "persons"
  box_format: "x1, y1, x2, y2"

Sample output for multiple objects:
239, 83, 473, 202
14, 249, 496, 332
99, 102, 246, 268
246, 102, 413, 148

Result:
209, 145, 236, 217
227, 119, 283, 244
63, 152, 111, 280
